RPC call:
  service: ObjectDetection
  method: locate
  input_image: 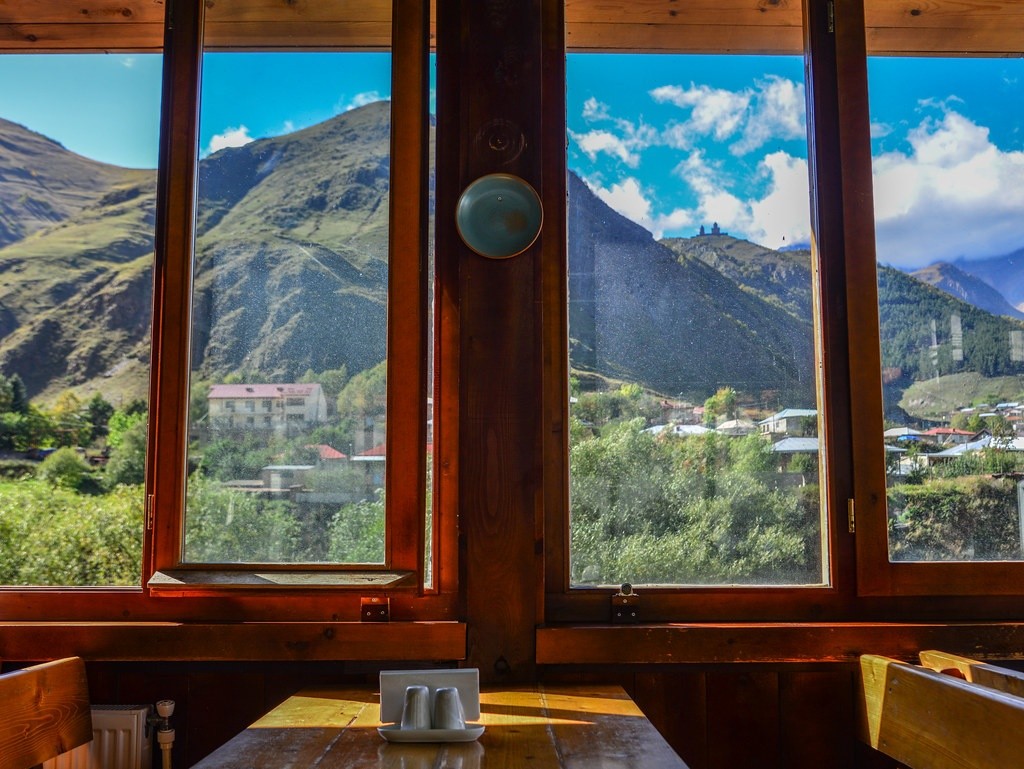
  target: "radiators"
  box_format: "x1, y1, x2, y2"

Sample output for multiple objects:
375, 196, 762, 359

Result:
43, 700, 175, 769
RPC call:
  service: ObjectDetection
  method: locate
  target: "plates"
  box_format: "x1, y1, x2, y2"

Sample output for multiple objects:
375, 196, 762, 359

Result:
377, 724, 485, 742
454, 173, 544, 259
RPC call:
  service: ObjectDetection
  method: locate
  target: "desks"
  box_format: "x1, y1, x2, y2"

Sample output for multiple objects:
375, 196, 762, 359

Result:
187, 676, 690, 769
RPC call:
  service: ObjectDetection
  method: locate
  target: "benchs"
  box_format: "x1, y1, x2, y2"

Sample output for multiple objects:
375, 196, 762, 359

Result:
0, 654, 93, 769
857, 648, 1024, 769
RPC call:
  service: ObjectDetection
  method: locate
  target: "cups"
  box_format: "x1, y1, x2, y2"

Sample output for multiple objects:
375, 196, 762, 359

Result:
401, 685, 431, 730
432, 687, 465, 730
